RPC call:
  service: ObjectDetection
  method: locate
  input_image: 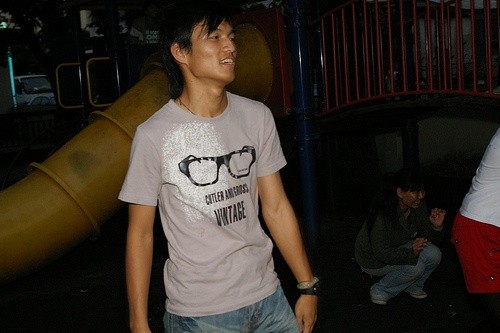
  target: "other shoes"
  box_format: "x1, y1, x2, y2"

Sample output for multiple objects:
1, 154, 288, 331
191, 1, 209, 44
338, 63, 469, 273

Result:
404, 289, 427, 298
372, 299, 387, 304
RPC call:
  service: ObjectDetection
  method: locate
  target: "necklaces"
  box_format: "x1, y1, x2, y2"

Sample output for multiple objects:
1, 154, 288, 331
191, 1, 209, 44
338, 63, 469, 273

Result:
178, 96, 196, 116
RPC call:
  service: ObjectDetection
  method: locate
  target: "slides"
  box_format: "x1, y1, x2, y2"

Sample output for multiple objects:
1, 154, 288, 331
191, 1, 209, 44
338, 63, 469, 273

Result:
0, 14, 280, 292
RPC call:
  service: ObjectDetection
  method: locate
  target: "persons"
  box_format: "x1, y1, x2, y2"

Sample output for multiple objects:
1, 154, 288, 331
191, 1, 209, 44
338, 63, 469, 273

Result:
353, 167, 446, 305
119, 1, 322, 333
452, 128, 500, 333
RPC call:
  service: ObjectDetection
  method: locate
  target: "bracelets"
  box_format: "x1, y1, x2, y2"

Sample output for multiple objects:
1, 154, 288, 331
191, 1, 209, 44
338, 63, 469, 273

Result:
296, 276, 320, 294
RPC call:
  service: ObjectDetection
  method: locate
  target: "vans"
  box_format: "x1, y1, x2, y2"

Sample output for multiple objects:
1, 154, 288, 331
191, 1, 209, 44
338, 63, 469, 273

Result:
13, 74, 56, 106
